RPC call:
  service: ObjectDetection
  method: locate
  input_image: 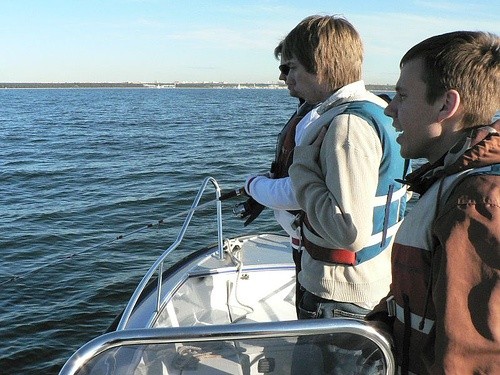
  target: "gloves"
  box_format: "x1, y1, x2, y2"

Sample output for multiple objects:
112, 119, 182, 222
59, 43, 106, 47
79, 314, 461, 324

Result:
235, 197, 266, 228
243, 174, 256, 195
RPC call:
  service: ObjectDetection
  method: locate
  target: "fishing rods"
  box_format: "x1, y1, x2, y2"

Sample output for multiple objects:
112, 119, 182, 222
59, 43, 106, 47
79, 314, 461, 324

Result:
0, 186, 246, 300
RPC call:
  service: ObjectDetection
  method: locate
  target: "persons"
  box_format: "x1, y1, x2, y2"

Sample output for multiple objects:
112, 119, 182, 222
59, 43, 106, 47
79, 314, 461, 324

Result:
235, 34, 316, 318
323, 31, 499, 375
287, 12, 408, 375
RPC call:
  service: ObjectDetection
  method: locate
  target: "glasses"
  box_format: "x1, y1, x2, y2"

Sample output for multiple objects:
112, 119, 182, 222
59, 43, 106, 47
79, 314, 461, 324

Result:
278, 64, 290, 76
